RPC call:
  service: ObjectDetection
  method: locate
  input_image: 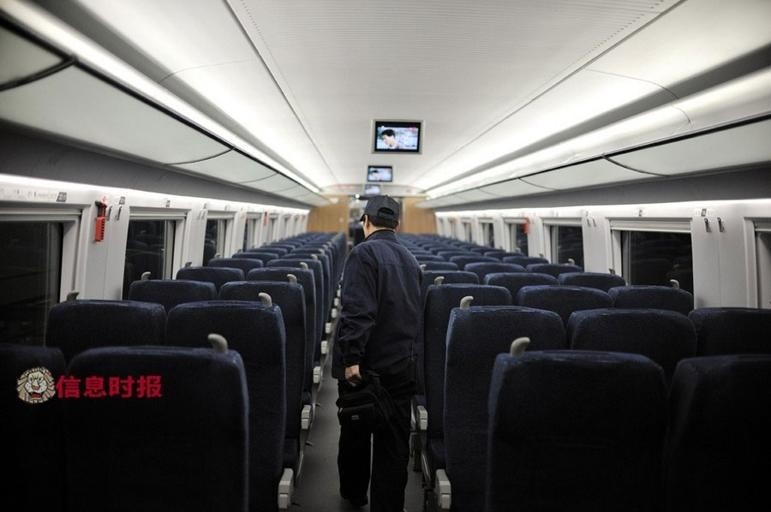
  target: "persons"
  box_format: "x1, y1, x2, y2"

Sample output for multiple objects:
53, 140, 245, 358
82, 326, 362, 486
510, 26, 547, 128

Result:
371, 169, 382, 180
332, 195, 424, 511
381, 130, 404, 149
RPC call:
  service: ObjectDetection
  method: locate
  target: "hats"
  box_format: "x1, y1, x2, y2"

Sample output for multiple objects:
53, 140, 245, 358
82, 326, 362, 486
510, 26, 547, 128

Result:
358, 195, 401, 222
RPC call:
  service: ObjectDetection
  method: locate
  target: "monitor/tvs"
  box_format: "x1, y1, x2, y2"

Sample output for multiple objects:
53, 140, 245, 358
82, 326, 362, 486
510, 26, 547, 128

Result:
367, 165, 393, 183
364, 184, 381, 197
373, 120, 422, 154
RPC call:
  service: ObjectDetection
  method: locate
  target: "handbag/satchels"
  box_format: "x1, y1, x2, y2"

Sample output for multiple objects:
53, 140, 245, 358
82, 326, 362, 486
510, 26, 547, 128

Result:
335, 368, 396, 434
